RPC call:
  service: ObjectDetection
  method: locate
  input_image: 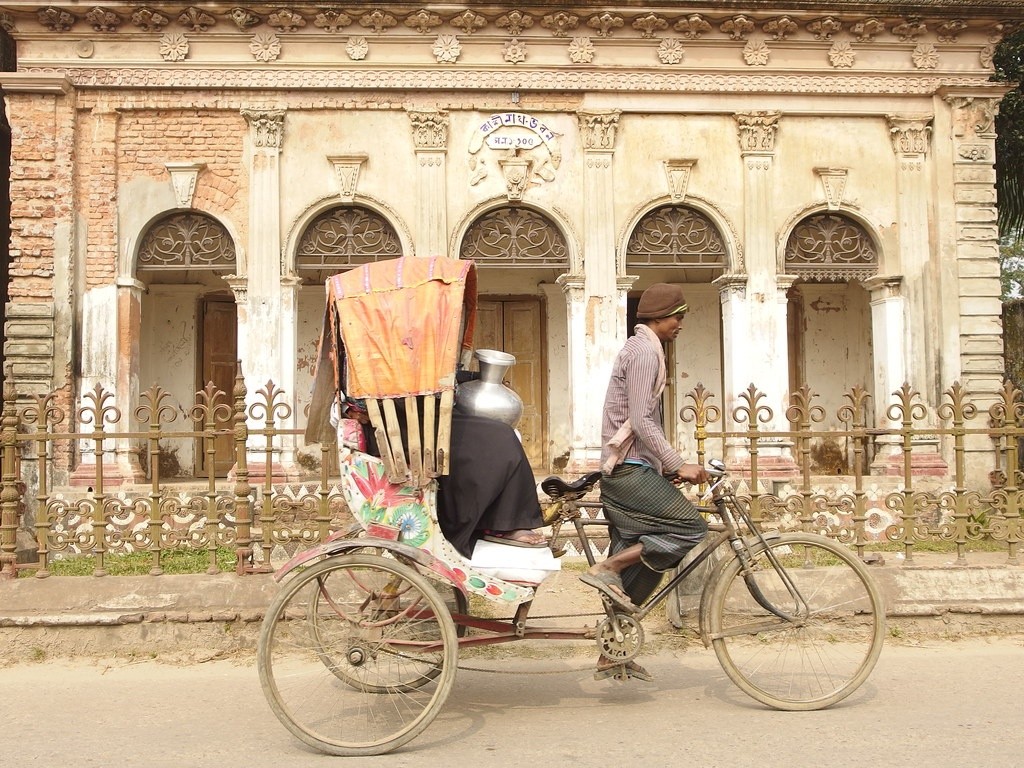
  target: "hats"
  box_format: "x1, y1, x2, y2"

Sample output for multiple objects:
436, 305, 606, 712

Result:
637, 282, 690, 319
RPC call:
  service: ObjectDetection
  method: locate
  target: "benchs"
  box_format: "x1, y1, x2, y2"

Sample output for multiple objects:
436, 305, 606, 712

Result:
337, 418, 438, 514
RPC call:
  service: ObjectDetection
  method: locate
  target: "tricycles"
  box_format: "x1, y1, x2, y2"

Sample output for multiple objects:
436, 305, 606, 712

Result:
254, 256, 886, 757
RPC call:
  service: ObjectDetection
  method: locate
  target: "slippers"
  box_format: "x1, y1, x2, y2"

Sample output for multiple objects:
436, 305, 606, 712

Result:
592, 659, 653, 682
579, 571, 638, 614
484, 528, 550, 549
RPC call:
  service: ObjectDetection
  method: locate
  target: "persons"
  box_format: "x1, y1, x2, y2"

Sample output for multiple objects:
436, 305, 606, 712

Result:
579, 282, 714, 680
341, 338, 548, 560
